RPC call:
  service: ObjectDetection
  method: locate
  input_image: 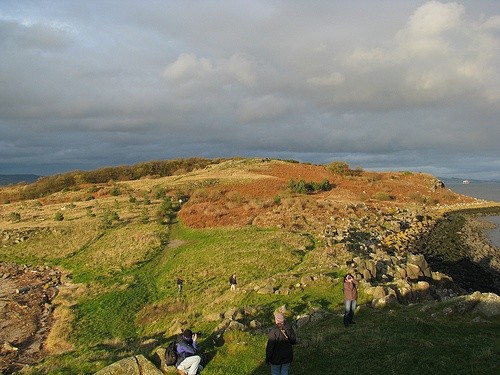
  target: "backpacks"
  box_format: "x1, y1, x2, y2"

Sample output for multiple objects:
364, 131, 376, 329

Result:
164, 341, 178, 366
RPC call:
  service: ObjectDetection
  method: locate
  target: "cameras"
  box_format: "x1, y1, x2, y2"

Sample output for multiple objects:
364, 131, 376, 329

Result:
347, 275, 351, 280
192, 331, 202, 338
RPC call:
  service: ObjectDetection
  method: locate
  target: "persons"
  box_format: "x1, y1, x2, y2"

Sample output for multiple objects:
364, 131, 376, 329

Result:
173, 329, 203, 375
229, 273, 237, 291
177, 276, 183, 293
178, 193, 190, 207
342, 273, 360, 329
265, 312, 296, 375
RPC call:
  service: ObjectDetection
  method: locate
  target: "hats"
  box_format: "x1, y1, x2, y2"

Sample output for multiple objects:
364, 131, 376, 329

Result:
275, 313, 285, 322
